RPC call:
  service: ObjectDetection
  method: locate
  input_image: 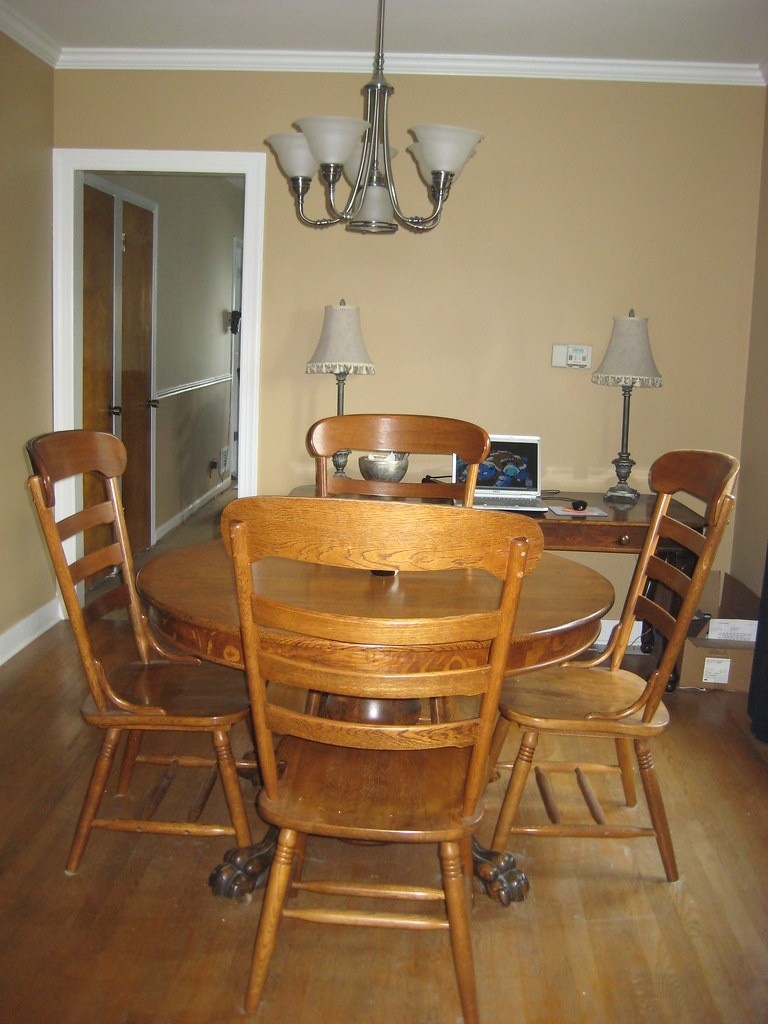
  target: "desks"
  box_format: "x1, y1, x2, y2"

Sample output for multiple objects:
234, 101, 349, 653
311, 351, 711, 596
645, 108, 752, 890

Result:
287, 485, 708, 693
134, 539, 615, 907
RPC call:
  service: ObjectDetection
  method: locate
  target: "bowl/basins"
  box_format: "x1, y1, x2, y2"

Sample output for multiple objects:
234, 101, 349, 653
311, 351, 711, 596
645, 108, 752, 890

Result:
358, 454, 408, 483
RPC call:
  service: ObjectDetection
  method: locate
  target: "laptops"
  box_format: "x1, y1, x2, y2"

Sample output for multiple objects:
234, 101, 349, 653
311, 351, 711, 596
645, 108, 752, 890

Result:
451, 435, 549, 515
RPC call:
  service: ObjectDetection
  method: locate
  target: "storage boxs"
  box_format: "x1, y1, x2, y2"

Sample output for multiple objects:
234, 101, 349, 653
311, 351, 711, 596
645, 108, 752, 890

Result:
652, 567, 761, 694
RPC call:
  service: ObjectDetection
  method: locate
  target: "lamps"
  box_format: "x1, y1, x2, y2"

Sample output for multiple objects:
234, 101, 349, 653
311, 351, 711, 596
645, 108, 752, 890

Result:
304, 298, 376, 477
263, 0, 483, 234
592, 308, 664, 501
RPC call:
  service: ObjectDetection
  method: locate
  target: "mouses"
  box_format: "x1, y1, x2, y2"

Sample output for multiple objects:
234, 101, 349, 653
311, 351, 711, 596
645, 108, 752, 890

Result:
572, 499, 587, 511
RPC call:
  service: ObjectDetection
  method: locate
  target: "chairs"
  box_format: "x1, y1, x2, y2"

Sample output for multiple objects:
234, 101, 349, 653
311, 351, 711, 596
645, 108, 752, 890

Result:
303, 413, 491, 724
24, 429, 253, 873
480, 450, 741, 882
222, 492, 543, 1022
